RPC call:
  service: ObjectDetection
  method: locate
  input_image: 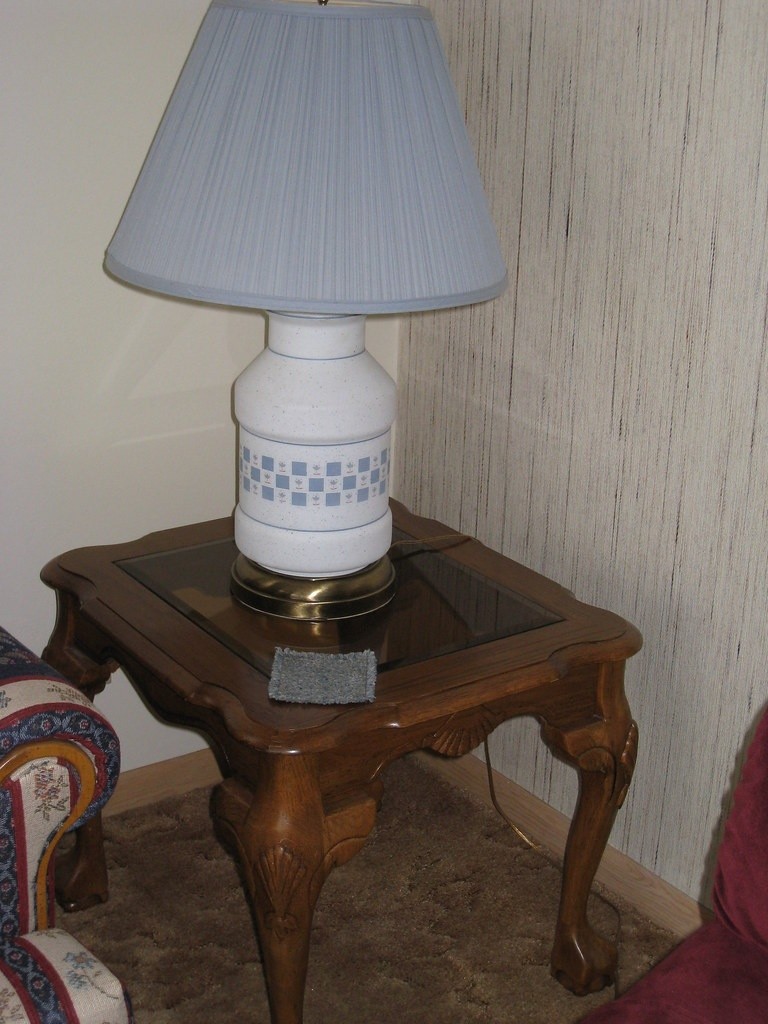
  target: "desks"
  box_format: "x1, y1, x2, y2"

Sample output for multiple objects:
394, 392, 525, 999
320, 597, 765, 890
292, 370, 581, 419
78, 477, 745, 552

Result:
38, 496, 642, 1024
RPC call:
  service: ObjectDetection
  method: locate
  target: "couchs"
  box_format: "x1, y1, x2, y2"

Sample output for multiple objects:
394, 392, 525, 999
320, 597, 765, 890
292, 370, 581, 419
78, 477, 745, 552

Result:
0, 627, 134, 1024
578, 708, 768, 1024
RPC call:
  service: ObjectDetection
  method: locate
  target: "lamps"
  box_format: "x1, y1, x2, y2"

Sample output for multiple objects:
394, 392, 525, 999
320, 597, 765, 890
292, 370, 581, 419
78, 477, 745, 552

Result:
104, 0, 508, 624
